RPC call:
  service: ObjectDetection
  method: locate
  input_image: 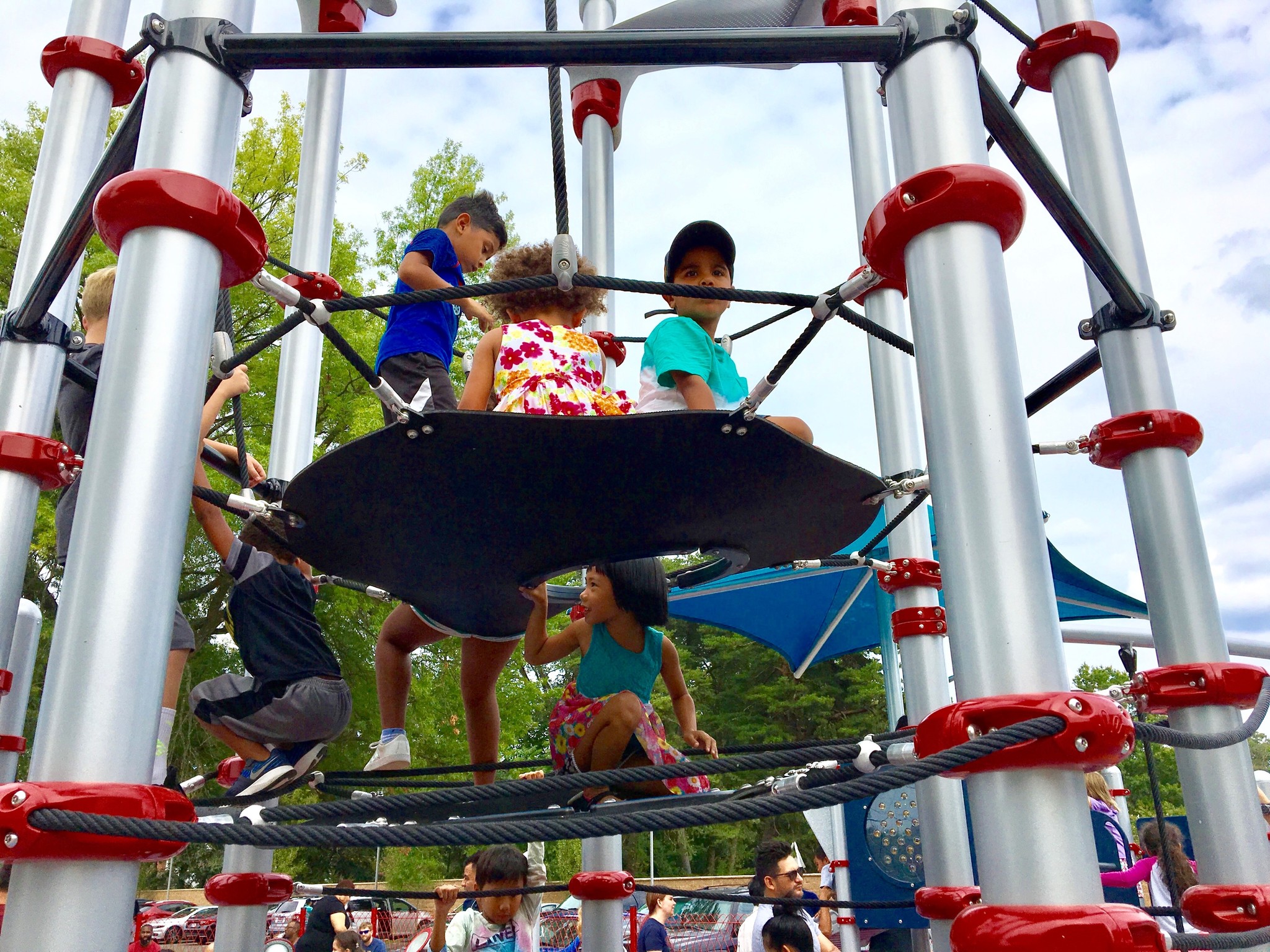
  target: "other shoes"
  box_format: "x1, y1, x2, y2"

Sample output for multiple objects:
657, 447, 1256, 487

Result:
583, 788, 622, 804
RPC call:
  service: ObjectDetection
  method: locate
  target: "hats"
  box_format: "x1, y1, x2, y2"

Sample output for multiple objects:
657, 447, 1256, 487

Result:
664, 220, 736, 286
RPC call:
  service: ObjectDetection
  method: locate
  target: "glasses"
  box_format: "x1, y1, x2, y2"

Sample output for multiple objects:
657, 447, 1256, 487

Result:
773, 867, 803, 881
359, 930, 371, 935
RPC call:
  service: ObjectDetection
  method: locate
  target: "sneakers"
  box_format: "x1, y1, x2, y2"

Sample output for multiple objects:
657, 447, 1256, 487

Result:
363, 734, 411, 771
218, 748, 298, 808
273, 741, 328, 791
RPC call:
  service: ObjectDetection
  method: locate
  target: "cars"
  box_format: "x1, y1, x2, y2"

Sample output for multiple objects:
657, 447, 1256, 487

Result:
141, 905, 220, 944
540, 884, 755, 952
265, 906, 277, 930
431, 903, 464, 927
133, 899, 198, 925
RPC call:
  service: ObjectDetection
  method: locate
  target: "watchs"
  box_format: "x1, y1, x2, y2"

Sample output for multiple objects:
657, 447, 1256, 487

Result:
1260, 803, 1270, 815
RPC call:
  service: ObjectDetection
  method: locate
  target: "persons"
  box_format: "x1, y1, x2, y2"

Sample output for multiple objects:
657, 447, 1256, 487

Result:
634, 220, 813, 445
1084, 771, 1144, 898
369, 189, 508, 426
273, 770, 914, 952
362, 601, 526, 788
1099, 821, 1213, 952
518, 556, 719, 804
54, 265, 267, 799
186, 364, 353, 796
1256, 785, 1270, 840
127, 899, 215, 952
455, 238, 637, 417
0, 864, 12, 935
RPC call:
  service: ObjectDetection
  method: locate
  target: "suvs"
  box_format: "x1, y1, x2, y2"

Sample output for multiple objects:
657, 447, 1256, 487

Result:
344, 896, 435, 938
268, 895, 325, 939
